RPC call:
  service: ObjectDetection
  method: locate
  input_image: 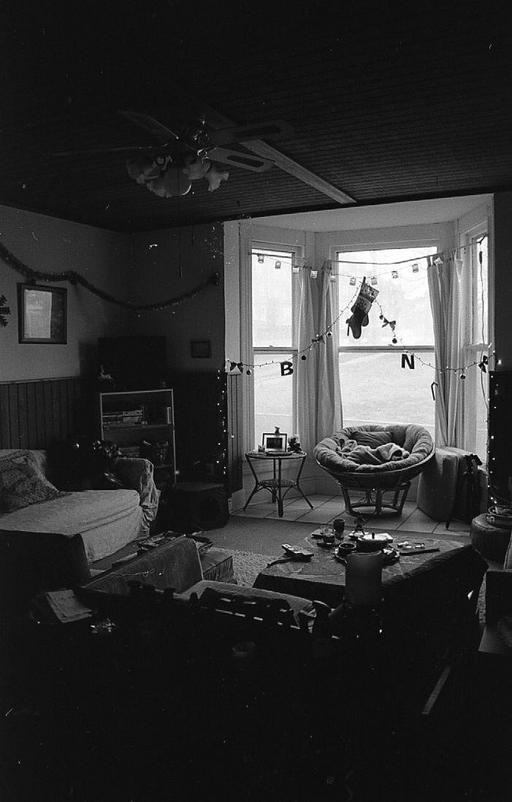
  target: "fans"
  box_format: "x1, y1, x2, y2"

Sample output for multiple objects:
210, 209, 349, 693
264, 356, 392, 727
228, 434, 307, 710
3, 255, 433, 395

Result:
44, 105, 275, 178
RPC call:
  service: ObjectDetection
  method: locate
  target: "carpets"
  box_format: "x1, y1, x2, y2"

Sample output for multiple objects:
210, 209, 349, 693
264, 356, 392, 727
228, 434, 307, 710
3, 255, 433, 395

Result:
95, 515, 508, 622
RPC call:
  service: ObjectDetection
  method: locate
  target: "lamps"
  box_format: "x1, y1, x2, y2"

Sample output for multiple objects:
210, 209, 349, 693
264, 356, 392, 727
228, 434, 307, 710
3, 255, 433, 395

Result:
118, 138, 233, 198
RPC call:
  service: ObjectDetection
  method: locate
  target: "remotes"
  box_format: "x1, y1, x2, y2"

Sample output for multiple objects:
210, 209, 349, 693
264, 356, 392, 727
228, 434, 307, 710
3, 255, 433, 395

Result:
282, 543, 302, 558
294, 546, 314, 558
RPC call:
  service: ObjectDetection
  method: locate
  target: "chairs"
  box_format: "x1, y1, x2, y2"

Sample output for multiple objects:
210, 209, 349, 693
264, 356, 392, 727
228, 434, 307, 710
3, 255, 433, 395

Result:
314, 421, 435, 521
0, 444, 156, 572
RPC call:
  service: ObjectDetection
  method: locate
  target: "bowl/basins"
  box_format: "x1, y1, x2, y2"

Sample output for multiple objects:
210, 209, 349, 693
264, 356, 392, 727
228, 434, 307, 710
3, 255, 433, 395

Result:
338, 541, 357, 553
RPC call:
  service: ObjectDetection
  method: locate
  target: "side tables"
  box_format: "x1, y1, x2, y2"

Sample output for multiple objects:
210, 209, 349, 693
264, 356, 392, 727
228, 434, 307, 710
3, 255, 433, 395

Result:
242, 452, 314, 517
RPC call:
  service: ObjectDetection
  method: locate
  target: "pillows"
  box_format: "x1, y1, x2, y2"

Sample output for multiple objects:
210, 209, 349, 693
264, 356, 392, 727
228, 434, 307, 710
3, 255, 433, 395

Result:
0, 451, 66, 513
351, 429, 393, 451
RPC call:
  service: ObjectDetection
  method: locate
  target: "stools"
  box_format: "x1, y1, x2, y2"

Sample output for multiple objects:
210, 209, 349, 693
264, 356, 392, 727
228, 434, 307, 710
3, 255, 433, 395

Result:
112, 537, 234, 587
163, 480, 230, 530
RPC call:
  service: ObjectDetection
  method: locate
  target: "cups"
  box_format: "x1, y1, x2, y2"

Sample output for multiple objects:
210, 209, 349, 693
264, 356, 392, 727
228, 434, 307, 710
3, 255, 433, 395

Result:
322, 518, 345, 544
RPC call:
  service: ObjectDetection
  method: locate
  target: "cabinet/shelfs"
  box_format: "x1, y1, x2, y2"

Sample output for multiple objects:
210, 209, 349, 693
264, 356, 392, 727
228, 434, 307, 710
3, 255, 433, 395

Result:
98, 388, 180, 483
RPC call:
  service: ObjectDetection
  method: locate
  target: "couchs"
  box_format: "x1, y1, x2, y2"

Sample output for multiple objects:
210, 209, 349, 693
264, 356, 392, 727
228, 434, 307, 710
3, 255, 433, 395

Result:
77, 537, 400, 746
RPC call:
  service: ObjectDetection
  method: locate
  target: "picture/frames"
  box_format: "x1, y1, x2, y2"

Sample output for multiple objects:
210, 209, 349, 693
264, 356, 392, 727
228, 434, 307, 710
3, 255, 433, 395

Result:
190, 339, 211, 360
262, 433, 287, 452
19, 282, 69, 346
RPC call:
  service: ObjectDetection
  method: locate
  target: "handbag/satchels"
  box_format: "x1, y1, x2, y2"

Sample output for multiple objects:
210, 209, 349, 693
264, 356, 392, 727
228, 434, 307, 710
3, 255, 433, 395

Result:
66, 441, 123, 490
